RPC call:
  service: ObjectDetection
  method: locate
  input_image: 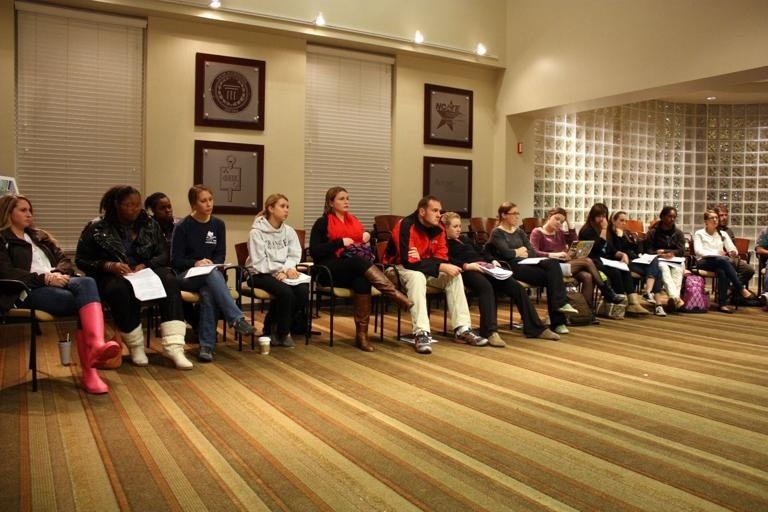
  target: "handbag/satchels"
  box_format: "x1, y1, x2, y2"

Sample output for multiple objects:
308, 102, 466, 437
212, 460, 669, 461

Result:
596, 293, 628, 320
564, 283, 593, 325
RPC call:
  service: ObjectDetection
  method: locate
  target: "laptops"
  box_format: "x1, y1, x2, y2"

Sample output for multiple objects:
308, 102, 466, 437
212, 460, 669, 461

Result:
551, 241, 595, 262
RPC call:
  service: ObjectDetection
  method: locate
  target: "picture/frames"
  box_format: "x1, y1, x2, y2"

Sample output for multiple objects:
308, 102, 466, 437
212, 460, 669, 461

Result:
194, 138, 264, 215
194, 52, 266, 131
423, 83, 477, 152
421, 155, 472, 220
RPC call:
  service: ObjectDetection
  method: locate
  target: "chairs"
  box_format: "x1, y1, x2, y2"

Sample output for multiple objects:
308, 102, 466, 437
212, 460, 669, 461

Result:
625, 216, 645, 235
755, 239, 768, 300
234, 242, 313, 346
372, 229, 456, 342
463, 272, 517, 332
579, 261, 613, 318
689, 236, 731, 311
294, 229, 308, 254
166, 251, 245, 356
512, 266, 578, 334
0, 272, 97, 393
462, 211, 540, 244
734, 236, 753, 294
629, 266, 649, 303
77, 261, 159, 351
369, 214, 402, 257
304, 236, 389, 348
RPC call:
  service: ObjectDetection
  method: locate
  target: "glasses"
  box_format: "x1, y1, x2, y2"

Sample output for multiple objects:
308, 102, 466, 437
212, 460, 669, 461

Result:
506, 212, 520, 215
120, 202, 143, 208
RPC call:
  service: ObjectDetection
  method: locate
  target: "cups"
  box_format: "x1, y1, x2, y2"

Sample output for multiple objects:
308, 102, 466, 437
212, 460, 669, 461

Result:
258, 336, 271, 355
56, 339, 72, 365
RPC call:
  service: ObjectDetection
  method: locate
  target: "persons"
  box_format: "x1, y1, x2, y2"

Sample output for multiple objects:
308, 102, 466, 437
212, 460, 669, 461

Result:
0, 195, 121, 395
244, 193, 312, 348
309, 186, 414, 353
74, 184, 257, 370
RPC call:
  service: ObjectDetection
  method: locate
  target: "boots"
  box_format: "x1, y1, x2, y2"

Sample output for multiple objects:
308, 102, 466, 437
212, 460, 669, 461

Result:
355, 295, 375, 352
76, 330, 109, 394
120, 323, 149, 367
627, 293, 650, 314
79, 302, 120, 368
598, 280, 624, 304
363, 265, 414, 311
160, 320, 193, 369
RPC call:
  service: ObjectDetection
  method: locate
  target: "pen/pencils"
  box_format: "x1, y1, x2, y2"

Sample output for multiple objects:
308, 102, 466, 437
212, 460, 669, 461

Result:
117, 256, 123, 264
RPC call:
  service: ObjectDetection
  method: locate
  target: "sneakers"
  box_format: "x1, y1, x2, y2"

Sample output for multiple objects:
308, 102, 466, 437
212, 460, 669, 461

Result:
486, 332, 506, 347
674, 297, 684, 309
655, 305, 666, 316
454, 325, 488, 346
761, 291, 768, 305
555, 324, 569, 334
198, 345, 213, 362
269, 334, 280, 346
559, 304, 579, 315
643, 293, 656, 304
282, 335, 295, 347
415, 331, 432, 354
233, 318, 257, 337
537, 328, 560, 340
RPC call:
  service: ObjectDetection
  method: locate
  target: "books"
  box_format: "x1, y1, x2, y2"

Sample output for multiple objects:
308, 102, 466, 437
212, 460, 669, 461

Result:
281, 272, 312, 287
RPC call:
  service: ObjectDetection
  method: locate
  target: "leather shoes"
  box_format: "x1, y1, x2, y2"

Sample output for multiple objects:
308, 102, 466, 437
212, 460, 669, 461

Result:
718, 306, 736, 314
739, 288, 754, 300
